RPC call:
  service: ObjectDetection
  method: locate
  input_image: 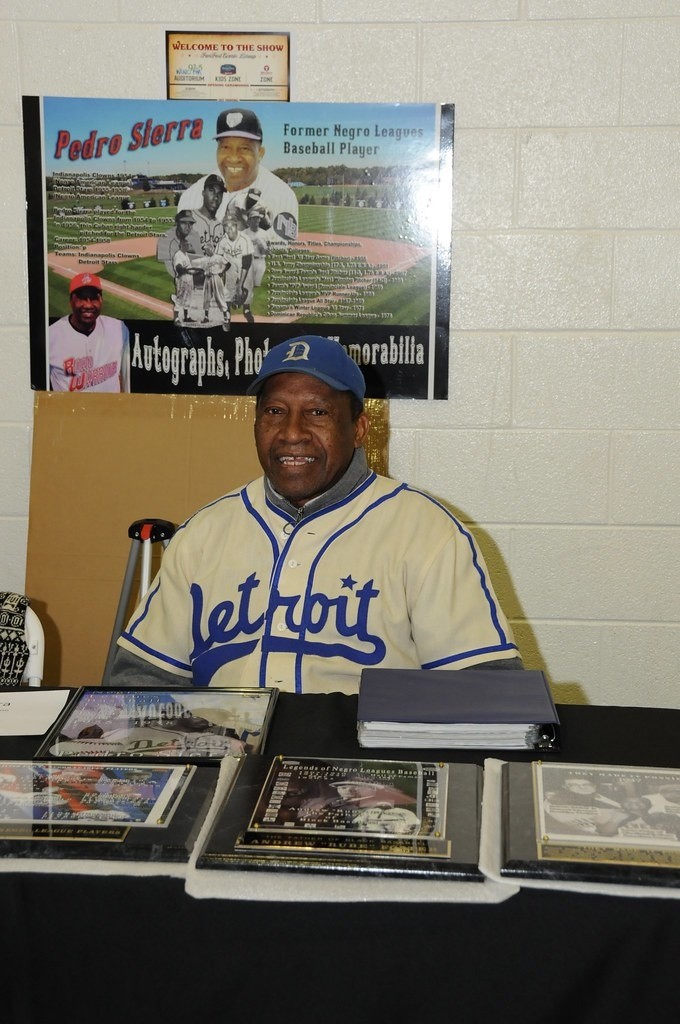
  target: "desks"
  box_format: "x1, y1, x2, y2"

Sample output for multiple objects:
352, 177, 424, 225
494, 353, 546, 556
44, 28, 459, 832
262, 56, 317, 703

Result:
1, 688, 680, 1024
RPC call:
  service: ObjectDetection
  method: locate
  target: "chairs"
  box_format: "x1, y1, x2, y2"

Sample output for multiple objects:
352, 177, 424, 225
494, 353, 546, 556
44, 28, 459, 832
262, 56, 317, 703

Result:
0, 592, 45, 687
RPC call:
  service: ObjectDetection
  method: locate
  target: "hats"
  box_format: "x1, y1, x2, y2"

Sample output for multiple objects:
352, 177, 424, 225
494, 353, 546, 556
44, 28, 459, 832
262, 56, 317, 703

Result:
203, 244, 213, 250
249, 211, 264, 219
222, 215, 237, 225
248, 188, 262, 201
203, 174, 228, 194
69, 272, 103, 291
247, 335, 366, 401
212, 108, 263, 141
175, 210, 196, 223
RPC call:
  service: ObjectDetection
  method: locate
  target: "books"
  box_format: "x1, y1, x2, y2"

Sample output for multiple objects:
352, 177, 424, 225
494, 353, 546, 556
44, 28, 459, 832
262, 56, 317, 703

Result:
358, 668, 560, 751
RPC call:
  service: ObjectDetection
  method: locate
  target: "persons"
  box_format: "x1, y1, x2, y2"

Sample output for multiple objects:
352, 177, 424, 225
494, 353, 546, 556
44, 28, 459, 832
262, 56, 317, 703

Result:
172, 108, 298, 332
108, 334, 522, 694
301, 772, 391, 827
50, 273, 129, 393
544, 777, 680, 845
